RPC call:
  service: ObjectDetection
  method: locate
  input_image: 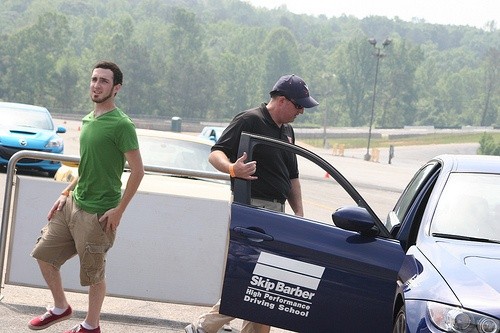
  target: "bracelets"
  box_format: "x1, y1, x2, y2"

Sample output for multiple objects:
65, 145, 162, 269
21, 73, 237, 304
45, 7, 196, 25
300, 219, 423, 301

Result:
62, 189, 69, 197
229, 164, 236, 178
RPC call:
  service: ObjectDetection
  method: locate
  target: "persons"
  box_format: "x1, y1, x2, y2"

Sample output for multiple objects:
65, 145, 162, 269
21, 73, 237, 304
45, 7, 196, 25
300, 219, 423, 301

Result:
185, 73, 320, 333
28, 61, 144, 333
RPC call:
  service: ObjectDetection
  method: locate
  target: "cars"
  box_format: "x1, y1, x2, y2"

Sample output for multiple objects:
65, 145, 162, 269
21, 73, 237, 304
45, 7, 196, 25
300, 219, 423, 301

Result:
218, 133, 500, 332
0, 102, 66, 175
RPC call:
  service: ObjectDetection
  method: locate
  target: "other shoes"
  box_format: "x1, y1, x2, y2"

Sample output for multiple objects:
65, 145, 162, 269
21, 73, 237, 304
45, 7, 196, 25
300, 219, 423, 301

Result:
184, 323, 199, 333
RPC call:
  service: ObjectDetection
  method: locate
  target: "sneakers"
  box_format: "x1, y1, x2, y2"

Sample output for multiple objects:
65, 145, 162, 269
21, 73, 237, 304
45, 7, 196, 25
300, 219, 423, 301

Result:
63, 324, 100, 333
28, 305, 72, 330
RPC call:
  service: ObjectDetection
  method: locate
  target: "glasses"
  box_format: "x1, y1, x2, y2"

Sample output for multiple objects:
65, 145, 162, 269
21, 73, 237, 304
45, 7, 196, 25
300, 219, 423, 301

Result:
280, 94, 304, 110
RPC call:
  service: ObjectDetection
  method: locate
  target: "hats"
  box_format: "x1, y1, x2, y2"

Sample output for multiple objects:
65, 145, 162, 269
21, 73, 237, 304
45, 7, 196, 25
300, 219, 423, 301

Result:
274, 74, 320, 108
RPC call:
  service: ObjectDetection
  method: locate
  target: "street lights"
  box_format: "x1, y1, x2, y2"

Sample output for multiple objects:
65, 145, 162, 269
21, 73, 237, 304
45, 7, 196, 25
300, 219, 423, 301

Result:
364, 37, 395, 159
